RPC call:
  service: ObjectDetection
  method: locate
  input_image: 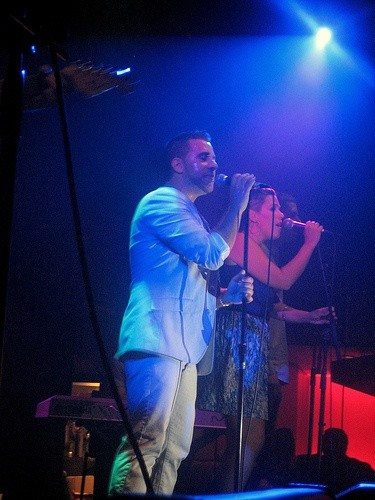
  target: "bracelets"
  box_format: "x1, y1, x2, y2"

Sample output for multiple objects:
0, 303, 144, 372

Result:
218, 294, 228, 308
281, 303, 289, 322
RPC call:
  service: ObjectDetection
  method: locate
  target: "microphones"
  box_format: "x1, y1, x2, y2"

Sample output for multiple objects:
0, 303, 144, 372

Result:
283, 218, 328, 233
214, 174, 270, 189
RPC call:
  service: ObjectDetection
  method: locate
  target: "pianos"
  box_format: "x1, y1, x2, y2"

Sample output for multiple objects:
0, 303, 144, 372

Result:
35, 392, 229, 433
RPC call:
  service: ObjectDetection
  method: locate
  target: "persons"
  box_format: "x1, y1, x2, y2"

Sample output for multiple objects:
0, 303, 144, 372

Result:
108, 129, 256, 496
195, 183, 375, 500
